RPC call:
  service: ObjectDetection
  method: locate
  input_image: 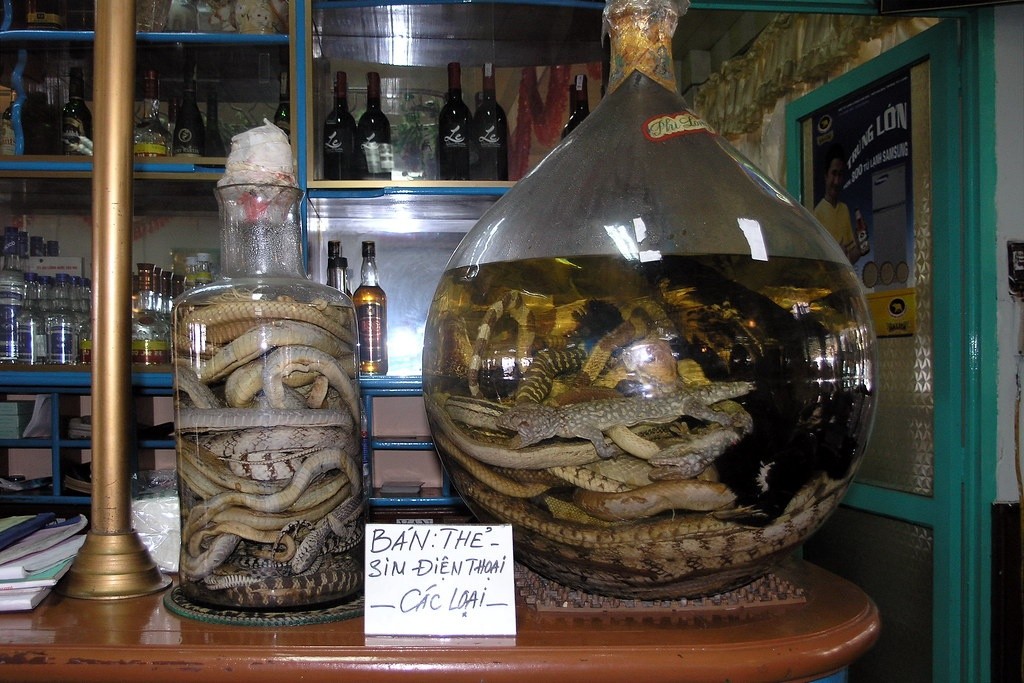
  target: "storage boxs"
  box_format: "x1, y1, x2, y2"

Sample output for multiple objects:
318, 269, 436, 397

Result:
0, 401, 35, 440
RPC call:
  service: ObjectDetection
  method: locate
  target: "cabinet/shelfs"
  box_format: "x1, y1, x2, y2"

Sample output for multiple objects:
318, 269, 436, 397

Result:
0, 17, 519, 515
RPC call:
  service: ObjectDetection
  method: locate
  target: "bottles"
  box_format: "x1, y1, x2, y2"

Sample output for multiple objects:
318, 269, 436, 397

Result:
561, 74, 591, 140
324, 240, 352, 300
134, 70, 226, 169
357, 71, 391, 180
438, 62, 473, 180
130, 253, 218, 368
168, 116, 366, 612
274, 71, 290, 145
360, 398, 371, 497
323, 71, 357, 180
472, 62, 509, 181
0, 226, 93, 366
61, 65, 92, 155
353, 240, 388, 376
422, 0, 876, 602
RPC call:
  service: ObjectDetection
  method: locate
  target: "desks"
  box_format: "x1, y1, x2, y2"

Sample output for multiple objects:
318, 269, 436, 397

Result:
0, 554, 880, 683
